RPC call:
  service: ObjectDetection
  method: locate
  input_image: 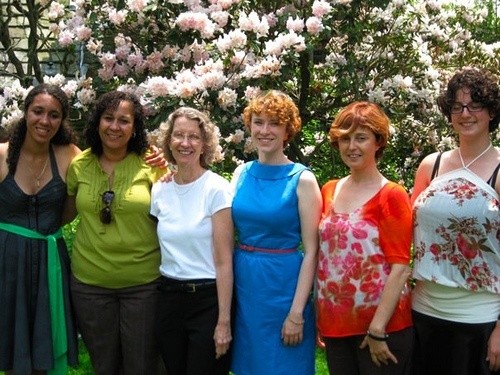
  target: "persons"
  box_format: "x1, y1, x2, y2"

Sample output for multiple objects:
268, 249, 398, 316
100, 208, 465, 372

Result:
159, 90, 324, 375
148, 107, 234, 375
0, 84, 170, 375
61, 91, 172, 375
410, 70, 500, 375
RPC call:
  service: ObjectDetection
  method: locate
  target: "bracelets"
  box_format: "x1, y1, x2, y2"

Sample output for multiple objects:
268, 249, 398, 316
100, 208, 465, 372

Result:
367, 331, 390, 341
288, 317, 305, 325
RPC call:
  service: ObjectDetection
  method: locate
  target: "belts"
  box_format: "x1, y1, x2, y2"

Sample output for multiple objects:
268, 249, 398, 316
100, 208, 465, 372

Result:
239, 245, 295, 253
163, 281, 216, 295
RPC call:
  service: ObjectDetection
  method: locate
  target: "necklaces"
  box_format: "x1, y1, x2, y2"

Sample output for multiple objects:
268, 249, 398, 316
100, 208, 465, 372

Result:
313, 100, 414, 375
20, 155, 49, 188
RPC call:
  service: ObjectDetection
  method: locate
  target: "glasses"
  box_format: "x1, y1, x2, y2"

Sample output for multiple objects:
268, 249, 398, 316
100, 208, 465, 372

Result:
100, 191, 113, 224
449, 102, 484, 114
